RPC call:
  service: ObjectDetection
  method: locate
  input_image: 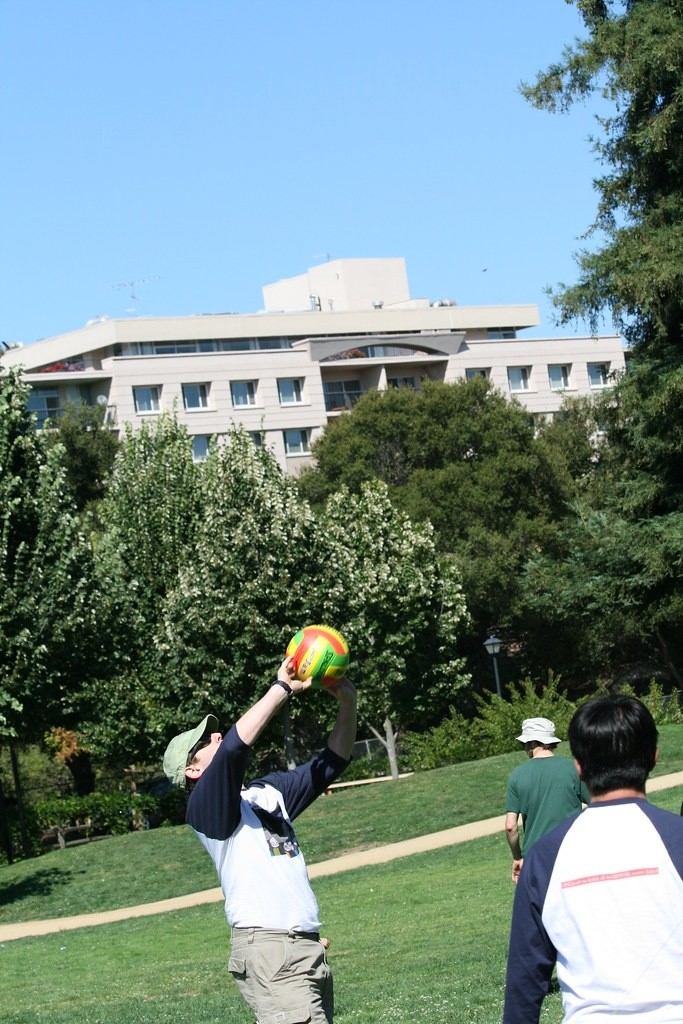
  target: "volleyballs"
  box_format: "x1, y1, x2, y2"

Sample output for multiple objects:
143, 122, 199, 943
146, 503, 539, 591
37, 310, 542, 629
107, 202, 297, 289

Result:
285, 622, 353, 688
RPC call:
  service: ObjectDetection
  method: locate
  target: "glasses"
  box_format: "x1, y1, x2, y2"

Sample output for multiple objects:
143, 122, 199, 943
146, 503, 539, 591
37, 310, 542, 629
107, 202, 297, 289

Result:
188, 736, 210, 767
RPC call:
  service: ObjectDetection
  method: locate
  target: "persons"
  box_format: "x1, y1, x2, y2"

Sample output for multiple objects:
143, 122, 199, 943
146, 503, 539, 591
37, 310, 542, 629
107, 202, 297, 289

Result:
162, 652, 361, 1024
501, 692, 683, 1024
505, 716, 593, 885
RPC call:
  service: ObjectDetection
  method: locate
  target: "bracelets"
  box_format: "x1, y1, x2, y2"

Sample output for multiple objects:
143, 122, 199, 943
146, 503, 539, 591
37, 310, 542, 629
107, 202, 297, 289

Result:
269, 679, 294, 696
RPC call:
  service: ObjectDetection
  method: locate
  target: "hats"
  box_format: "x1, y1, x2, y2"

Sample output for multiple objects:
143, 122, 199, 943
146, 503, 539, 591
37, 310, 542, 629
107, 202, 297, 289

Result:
163, 714, 219, 793
516, 718, 561, 744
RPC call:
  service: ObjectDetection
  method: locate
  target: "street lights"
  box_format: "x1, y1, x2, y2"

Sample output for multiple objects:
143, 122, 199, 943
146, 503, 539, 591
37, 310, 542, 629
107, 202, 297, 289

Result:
482, 635, 503, 697
